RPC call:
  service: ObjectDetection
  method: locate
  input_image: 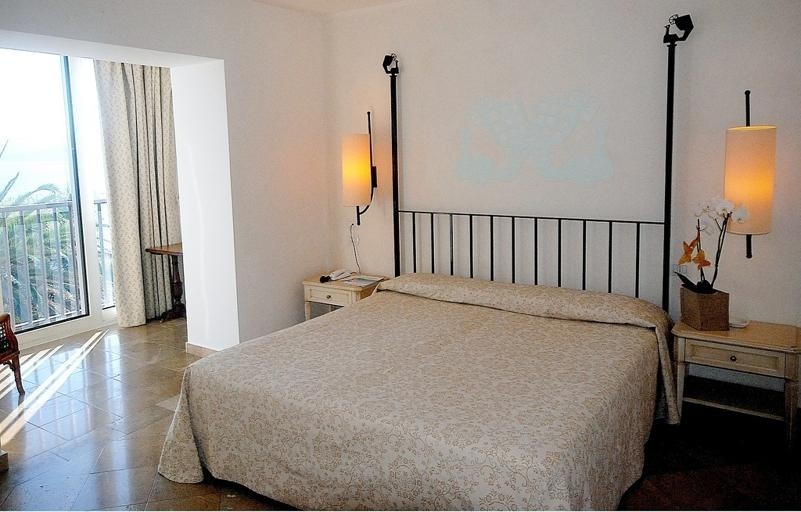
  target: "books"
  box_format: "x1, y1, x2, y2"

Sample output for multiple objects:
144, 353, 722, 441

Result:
341, 274, 385, 288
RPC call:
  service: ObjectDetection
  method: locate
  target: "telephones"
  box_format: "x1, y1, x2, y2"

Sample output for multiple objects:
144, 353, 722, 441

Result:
330, 267, 351, 281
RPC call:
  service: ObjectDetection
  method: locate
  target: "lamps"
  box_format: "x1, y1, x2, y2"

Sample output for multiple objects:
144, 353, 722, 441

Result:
725, 90, 777, 258
339, 111, 380, 226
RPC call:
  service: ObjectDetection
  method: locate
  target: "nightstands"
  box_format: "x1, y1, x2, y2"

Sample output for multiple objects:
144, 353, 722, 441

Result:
301, 273, 391, 321
675, 307, 801, 435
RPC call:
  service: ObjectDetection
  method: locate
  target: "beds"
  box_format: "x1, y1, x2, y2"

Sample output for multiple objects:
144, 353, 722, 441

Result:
186, 274, 662, 510
